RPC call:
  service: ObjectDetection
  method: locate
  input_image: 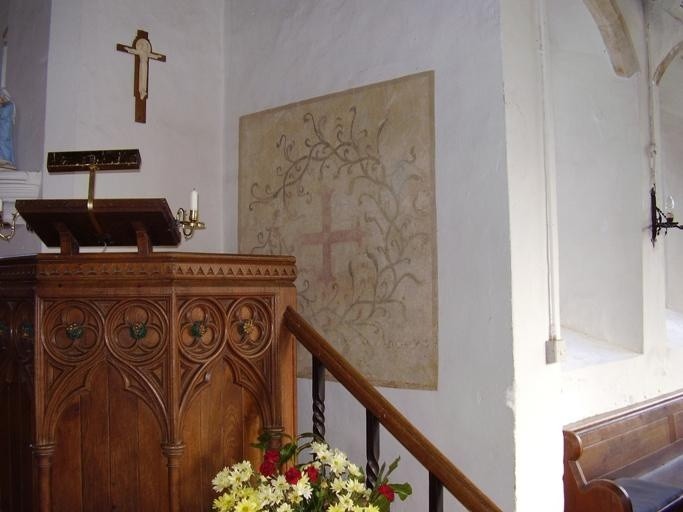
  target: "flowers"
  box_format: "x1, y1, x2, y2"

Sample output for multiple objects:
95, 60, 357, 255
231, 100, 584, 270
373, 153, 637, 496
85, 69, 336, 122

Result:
211, 431, 412, 512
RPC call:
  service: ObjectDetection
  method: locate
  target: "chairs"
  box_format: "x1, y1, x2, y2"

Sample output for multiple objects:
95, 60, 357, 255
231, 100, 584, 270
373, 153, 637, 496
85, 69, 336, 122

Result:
562, 429, 683, 512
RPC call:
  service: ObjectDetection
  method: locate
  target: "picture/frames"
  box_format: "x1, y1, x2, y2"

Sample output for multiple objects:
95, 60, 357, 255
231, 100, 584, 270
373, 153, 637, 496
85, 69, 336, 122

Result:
237, 69, 438, 391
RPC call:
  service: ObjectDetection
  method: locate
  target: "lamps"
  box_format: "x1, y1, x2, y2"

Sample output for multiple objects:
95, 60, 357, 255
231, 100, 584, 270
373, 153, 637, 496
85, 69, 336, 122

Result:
652, 190, 683, 241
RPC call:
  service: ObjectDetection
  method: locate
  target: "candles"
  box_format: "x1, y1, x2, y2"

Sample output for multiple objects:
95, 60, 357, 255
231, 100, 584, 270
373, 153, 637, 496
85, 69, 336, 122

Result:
0, 41, 8, 89
190, 189, 198, 221
